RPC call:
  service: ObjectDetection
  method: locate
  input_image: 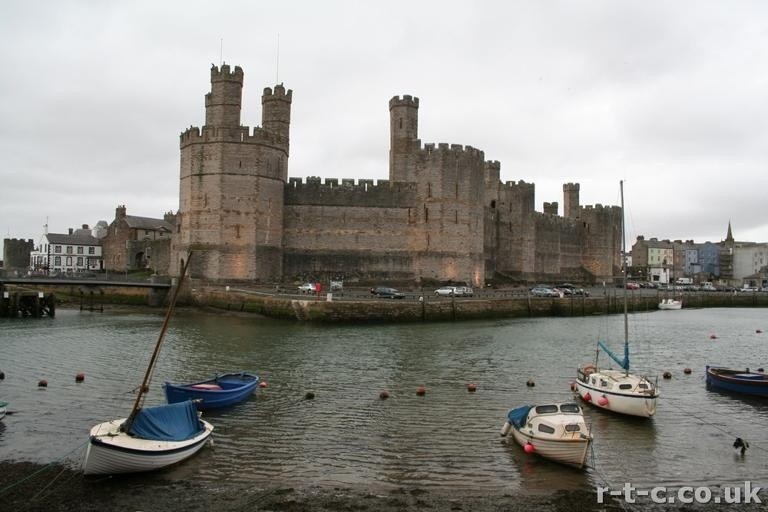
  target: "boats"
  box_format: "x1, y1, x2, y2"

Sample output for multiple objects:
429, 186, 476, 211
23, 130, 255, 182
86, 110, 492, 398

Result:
705, 365, 768, 398
499, 402, 594, 471
0, 401, 9, 421
80, 371, 259, 476
657, 299, 682, 309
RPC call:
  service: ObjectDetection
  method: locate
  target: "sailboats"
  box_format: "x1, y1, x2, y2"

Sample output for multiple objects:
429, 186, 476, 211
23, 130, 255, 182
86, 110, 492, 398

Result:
574, 181, 660, 420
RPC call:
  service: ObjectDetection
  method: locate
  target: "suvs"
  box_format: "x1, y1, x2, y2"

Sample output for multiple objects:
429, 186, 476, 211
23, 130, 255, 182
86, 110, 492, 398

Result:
374, 286, 406, 299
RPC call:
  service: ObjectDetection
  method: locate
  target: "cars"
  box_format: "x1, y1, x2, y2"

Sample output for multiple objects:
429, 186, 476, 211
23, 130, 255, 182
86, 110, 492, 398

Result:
626, 281, 768, 292
297, 283, 317, 295
530, 282, 584, 298
434, 285, 475, 298
